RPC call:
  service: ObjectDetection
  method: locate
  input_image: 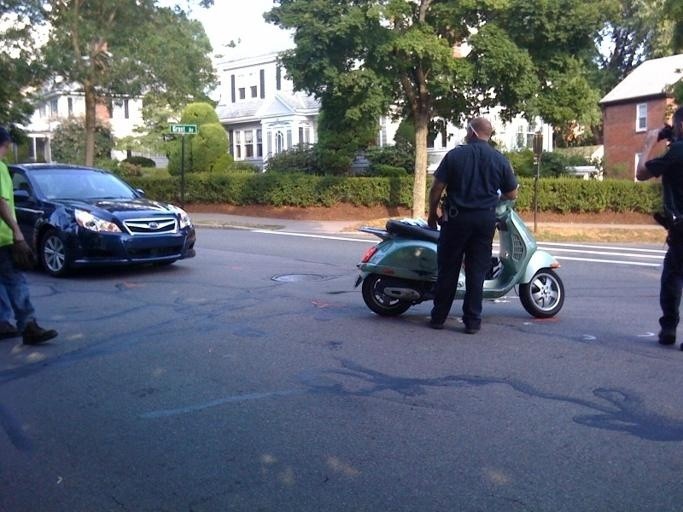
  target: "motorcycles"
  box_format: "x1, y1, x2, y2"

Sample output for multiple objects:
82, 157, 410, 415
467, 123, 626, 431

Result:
355, 187, 564, 318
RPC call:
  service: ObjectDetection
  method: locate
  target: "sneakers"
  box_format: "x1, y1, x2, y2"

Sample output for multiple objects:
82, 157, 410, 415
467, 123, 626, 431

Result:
429, 318, 444, 330
0, 321, 23, 338
466, 320, 482, 332
16, 318, 59, 345
657, 328, 676, 345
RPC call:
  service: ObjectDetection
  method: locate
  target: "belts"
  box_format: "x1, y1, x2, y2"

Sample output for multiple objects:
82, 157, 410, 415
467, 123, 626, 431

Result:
446, 202, 496, 214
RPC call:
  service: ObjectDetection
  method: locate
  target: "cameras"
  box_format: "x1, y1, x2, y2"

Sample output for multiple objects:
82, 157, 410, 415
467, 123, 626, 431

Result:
658, 123, 672, 141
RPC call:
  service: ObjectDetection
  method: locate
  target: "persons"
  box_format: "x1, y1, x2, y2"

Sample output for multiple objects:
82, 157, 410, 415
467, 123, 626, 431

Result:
636, 106, 683, 348
0, 127, 59, 345
427, 117, 518, 335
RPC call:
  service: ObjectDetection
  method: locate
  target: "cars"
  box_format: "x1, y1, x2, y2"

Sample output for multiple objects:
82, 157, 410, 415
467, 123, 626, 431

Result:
8, 163, 195, 277
